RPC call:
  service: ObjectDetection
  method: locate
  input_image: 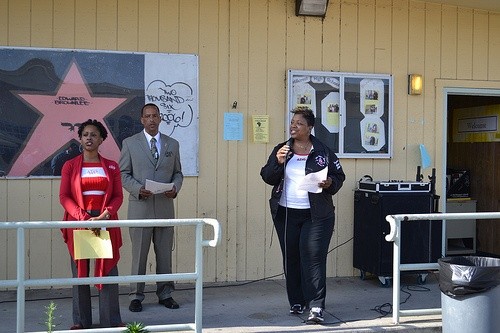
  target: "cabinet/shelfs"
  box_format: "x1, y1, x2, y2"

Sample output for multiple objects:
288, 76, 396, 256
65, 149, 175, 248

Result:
447, 199, 478, 255
352, 190, 438, 275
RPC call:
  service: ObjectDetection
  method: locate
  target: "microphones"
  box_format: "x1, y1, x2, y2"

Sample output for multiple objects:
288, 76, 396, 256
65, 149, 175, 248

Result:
284, 141, 292, 162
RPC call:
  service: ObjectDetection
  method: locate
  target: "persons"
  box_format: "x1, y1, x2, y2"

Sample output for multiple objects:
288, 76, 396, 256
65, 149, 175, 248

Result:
60, 119, 123, 330
260, 105, 346, 321
119, 103, 184, 312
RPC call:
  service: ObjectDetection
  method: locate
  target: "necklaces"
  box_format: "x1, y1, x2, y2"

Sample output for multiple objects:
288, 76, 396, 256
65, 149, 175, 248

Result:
297, 143, 309, 151
85, 157, 97, 160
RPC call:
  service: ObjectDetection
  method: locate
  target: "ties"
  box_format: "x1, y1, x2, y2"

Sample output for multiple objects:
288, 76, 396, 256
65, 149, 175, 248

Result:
150, 138, 159, 161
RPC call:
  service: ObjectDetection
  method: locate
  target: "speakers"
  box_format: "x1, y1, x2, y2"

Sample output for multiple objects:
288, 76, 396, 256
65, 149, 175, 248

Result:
353, 191, 432, 277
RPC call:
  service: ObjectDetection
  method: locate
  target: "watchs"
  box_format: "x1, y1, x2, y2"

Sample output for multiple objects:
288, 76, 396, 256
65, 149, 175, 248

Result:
104, 211, 110, 218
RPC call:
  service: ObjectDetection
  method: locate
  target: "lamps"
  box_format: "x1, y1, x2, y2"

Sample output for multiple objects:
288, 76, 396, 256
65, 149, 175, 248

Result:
408, 73, 423, 95
295, 0, 330, 18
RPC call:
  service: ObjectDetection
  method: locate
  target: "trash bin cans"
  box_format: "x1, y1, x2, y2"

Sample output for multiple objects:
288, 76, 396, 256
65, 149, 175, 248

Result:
438, 255, 500, 333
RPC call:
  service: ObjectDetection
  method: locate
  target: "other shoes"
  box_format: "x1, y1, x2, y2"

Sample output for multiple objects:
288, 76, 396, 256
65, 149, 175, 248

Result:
158, 298, 178, 310
290, 303, 306, 313
128, 299, 142, 312
308, 306, 324, 321
